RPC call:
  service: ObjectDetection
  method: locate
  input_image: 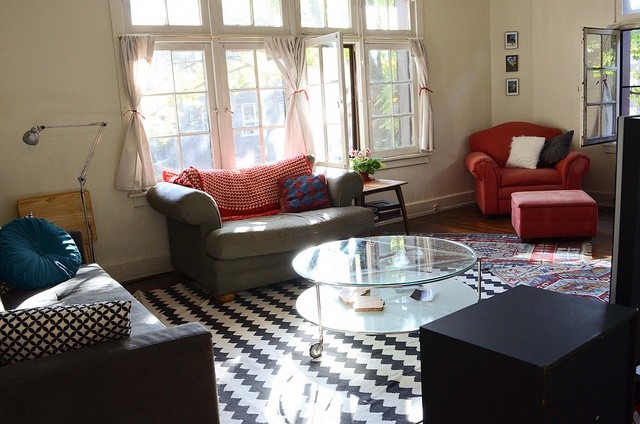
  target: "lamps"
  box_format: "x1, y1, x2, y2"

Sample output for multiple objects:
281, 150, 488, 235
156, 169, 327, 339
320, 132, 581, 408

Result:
23, 121, 106, 263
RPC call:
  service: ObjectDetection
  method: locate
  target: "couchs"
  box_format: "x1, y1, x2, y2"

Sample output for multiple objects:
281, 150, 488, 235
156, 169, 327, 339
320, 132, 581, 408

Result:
146, 152, 375, 301
464, 121, 593, 215
0, 218, 221, 424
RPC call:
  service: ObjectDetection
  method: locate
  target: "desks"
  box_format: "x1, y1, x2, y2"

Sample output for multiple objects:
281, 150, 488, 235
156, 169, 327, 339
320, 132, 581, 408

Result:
356, 179, 411, 232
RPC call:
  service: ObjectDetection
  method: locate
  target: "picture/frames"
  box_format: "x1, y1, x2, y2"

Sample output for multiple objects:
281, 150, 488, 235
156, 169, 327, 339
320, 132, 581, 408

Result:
505, 55, 518, 73
505, 77, 520, 96
504, 31, 518, 50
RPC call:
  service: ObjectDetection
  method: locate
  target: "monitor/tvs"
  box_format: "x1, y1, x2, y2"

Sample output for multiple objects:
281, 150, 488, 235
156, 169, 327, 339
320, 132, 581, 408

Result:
610, 116, 634, 304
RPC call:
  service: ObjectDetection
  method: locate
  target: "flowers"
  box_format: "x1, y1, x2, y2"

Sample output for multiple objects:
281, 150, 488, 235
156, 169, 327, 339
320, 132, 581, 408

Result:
348, 149, 381, 183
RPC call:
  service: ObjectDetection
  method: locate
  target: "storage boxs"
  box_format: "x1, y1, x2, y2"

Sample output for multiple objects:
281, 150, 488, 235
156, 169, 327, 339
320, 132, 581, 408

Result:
419, 284, 640, 423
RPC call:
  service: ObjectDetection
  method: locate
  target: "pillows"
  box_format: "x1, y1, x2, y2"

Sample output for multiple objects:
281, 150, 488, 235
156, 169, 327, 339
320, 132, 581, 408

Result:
162, 152, 312, 222
506, 134, 546, 169
0, 301, 133, 364
538, 130, 574, 168
277, 173, 330, 212
0, 218, 82, 290
